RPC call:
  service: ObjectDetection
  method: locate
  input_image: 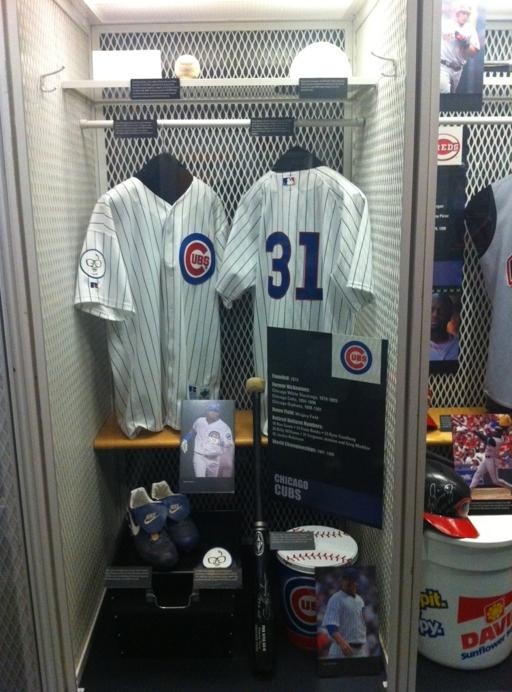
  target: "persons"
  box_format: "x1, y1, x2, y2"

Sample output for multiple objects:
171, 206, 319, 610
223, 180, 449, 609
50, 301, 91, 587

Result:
440, 4, 480, 94
180, 402, 235, 478
452, 415, 512, 495
321, 570, 369, 658
430, 292, 458, 361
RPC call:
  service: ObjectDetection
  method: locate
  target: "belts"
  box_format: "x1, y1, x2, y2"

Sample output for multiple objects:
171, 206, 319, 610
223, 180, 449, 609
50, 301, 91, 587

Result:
442, 59, 460, 72
195, 452, 216, 459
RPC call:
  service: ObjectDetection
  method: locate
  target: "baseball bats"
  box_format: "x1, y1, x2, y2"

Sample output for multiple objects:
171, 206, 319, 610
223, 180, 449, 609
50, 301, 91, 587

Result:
245, 376, 278, 683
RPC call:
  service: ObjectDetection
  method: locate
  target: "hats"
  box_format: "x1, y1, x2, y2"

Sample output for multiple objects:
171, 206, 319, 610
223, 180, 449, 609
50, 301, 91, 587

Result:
207, 402, 222, 412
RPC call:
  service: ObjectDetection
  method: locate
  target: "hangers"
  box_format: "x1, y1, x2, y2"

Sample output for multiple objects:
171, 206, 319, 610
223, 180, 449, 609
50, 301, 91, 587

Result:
126, 127, 191, 189
269, 120, 324, 173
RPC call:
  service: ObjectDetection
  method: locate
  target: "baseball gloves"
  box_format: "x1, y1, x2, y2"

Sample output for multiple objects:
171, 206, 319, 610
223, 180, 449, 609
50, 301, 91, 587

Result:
455, 31, 469, 46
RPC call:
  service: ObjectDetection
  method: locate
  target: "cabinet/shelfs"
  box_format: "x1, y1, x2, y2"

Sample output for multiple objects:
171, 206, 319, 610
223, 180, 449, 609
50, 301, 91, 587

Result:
62, 72, 370, 451
422, 60, 512, 445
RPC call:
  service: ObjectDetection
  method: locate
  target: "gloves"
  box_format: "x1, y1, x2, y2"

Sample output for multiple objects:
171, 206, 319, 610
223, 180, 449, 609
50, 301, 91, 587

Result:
180, 438, 188, 454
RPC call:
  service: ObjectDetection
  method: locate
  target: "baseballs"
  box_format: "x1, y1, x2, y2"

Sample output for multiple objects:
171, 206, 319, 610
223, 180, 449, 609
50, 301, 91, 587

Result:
174, 55, 200, 78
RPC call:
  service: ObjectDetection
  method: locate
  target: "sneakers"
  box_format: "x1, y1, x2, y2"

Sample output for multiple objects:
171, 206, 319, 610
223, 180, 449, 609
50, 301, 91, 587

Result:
150, 480, 200, 549
128, 486, 176, 568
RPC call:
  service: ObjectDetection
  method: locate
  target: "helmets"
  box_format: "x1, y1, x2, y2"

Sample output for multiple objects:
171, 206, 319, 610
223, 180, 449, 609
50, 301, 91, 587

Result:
424, 459, 481, 538
